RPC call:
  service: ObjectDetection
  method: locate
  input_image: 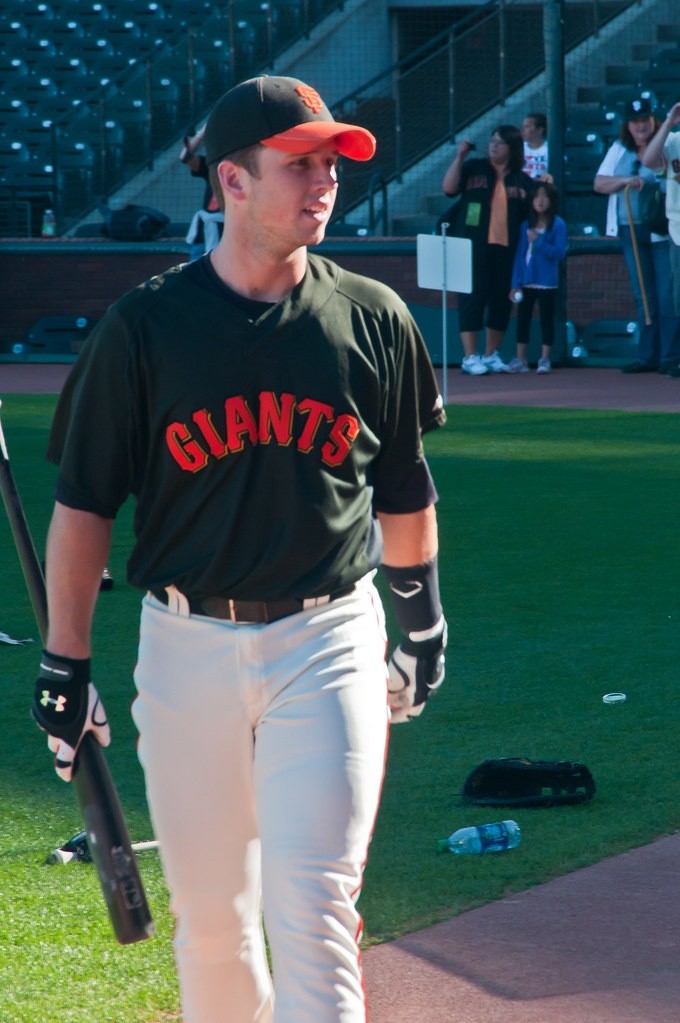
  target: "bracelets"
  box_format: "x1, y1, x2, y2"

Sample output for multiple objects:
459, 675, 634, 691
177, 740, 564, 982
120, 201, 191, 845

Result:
196, 129, 206, 140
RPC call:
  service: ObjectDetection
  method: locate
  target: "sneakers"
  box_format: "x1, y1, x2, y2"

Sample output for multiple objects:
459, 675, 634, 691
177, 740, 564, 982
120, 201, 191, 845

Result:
506, 357, 529, 373
482, 351, 511, 373
537, 358, 551, 374
460, 353, 489, 375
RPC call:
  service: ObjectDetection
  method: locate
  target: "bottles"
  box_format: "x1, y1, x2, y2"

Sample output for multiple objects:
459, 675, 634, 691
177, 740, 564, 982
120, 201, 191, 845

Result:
434, 820, 521, 857
41, 209, 56, 239
510, 290, 523, 304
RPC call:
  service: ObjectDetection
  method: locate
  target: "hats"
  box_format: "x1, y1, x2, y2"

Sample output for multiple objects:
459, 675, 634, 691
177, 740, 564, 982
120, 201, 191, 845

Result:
626, 99, 652, 120
204, 75, 377, 165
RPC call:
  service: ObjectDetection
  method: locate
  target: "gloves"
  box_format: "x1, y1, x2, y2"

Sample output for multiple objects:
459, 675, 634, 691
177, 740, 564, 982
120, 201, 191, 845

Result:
384, 613, 451, 724
31, 651, 111, 782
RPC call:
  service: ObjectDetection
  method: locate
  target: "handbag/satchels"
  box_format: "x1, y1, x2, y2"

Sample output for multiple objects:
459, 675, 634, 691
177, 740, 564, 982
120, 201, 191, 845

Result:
638, 182, 668, 236
433, 158, 480, 238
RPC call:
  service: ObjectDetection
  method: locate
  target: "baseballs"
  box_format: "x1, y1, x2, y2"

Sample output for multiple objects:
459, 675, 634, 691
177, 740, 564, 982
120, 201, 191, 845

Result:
512, 291, 523, 303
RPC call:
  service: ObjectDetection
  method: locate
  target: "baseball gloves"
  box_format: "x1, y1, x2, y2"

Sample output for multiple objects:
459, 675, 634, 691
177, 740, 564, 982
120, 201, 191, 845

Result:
461, 758, 597, 807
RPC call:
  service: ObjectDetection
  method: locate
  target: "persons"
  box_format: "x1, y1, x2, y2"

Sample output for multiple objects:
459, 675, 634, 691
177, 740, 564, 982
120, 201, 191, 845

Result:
29, 74, 450, 1023
444, 124, 554, 376
593, 96, 676, 375
641, 102, 680, 377
521, 113, 548, 180
178, 123, 225, 262
498, 180, 569, 374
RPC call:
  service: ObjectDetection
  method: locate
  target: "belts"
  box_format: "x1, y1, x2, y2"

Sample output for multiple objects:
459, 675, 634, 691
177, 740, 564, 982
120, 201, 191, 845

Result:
147, 583, 355, 624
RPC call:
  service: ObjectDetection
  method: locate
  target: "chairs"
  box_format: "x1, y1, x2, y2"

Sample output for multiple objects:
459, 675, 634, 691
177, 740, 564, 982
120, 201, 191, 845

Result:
0, 0, 336, 238
389, 19, 680, 238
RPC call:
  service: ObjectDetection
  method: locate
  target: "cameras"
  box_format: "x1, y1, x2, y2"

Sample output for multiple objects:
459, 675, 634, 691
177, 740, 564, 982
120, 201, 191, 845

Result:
468, 142, 476, 152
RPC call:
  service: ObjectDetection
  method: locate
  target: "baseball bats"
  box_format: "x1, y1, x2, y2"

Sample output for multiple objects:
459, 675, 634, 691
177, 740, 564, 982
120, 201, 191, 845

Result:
0, 400, 157, 945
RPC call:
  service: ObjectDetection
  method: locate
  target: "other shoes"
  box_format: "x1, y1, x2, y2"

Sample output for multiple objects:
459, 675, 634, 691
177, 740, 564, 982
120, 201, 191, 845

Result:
623, 362, 658, 372
659, 362, 680, 378
